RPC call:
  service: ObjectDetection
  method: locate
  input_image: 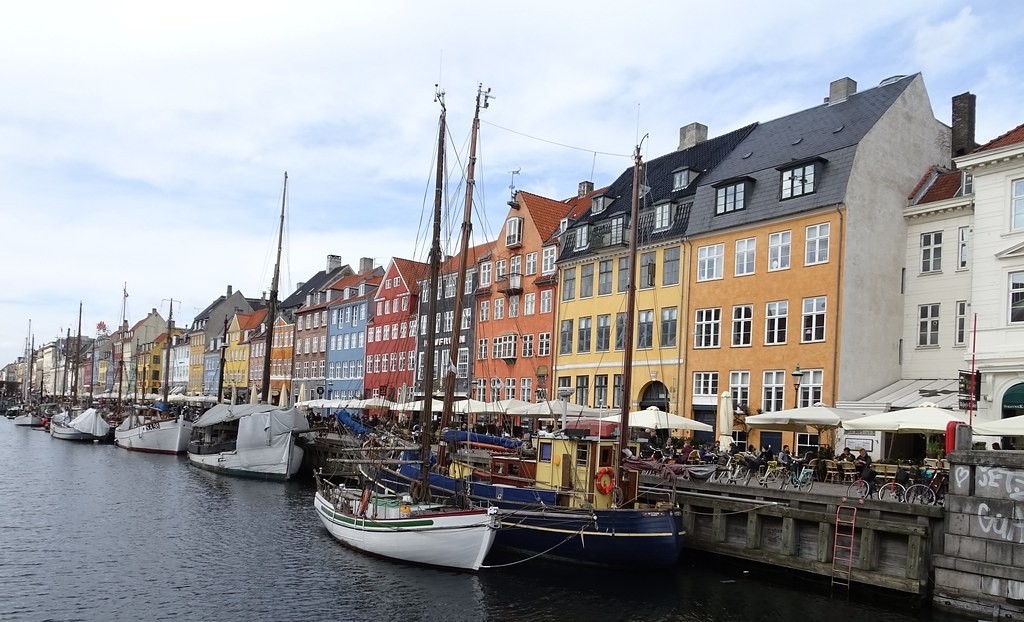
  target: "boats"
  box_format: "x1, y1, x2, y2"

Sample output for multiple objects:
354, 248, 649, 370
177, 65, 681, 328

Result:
1, 79, 692, 581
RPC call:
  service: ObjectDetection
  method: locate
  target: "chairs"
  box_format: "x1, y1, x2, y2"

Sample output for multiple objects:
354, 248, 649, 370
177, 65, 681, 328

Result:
306, 390, 957, 528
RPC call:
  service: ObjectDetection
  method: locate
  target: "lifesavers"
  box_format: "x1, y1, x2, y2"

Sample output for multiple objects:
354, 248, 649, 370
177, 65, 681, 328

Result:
358, 489, 370, 517
596, 468, 616, 494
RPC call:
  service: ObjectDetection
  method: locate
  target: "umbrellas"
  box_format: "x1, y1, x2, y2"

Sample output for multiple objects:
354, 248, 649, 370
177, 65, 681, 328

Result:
972, 414, 1024, 436
745, 402, 870, 451
720, 391, 736, 451
47, 382, 606, 419
842, 402, 965, 462
598, 405, 714, 433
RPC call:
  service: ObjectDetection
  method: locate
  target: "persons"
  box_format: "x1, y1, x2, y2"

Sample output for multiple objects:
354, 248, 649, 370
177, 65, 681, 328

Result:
728, 443, 740, 457
663, 438, 677, 457
747, 444, 761, 457
837, 447, 855, 481
854, 448, 872, 478
306, 413, 528, 434
700, 444, 713, 463
778, 445, 795, 471
677, 441, 693, 464
992, 442, 1002, 450
761, 444, 774, 476
709, 441, 720, 453
799, 448, 814, 470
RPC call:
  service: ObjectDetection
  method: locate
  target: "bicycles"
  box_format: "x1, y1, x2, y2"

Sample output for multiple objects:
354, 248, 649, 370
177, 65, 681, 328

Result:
706, 443, 950, 508
